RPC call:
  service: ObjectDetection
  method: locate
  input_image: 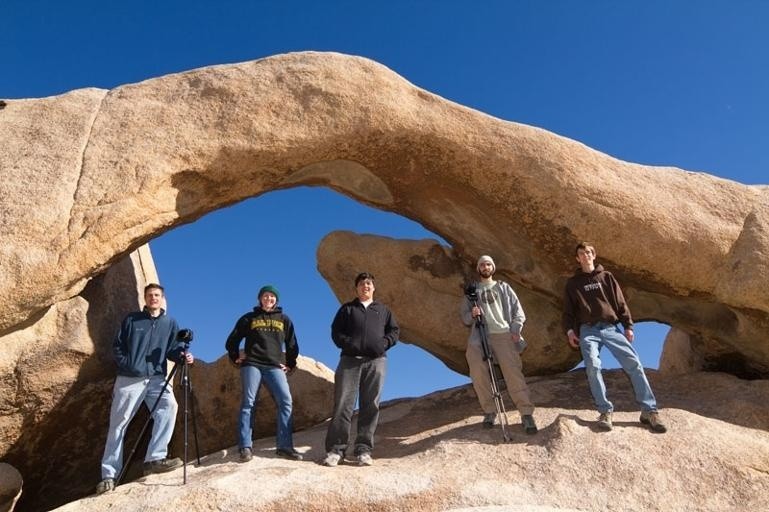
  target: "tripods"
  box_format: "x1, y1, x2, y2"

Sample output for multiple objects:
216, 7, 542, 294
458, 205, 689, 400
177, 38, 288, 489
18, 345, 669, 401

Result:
469, 294, 513, 443
112, 356, 200, 492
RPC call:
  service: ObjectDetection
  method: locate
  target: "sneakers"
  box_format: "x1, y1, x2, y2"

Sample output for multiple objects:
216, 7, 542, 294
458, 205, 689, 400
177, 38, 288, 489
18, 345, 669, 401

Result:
323, 452, 344, 466
240, 448, 252, 461
96, 478, 115, 493
599, 412, 611, 428
356, 453, 372, 466
143, 457, 184, 475
276, 447, 304, 461
483, 413, 495, 428
522, 415, 537, 433
640, 410, 666, 433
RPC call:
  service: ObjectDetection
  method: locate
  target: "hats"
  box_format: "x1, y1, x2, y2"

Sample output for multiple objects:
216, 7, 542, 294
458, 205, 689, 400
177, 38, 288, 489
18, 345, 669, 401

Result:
258, 285, 279, 302
477, 255, 495, 274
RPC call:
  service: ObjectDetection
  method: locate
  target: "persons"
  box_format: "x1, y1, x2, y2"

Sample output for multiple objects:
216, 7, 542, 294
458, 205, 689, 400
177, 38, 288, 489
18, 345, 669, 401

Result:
461, 255, 537, 435
225, 285, 305, 462
96, 283, 194, 495
322, 270, 400, 466
560, 242, 667, 432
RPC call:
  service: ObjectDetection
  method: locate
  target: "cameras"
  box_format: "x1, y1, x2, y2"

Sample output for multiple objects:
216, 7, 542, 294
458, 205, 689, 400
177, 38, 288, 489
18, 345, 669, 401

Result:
464, 279, 476, 295
176, 328, 193, 343
